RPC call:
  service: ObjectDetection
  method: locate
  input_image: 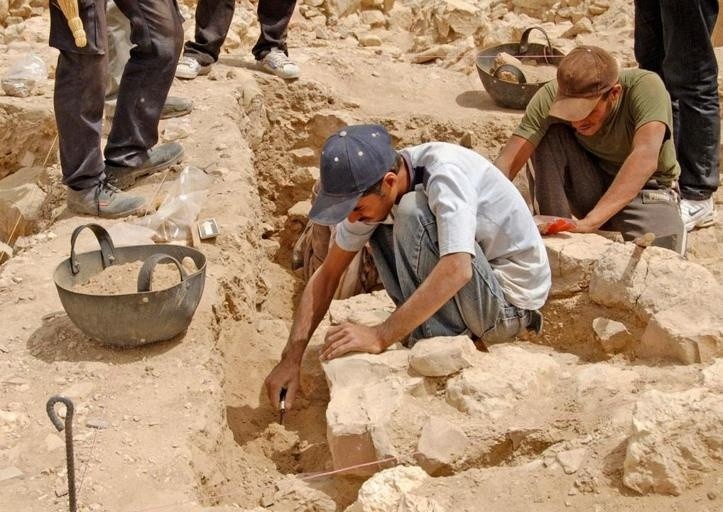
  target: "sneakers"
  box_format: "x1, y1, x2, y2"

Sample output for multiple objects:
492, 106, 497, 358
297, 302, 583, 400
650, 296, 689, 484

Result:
173, 54, 214, 78
66, 182, 153, 219
680, 194, 717, 230
102, 143, 183, 189
255, 49, 301, 82
162, 95, 193, 116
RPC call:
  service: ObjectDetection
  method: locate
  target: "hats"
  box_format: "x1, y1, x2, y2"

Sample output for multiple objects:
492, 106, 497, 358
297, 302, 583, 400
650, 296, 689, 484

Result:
308, 123, 397, 227
547, 44, 620, 124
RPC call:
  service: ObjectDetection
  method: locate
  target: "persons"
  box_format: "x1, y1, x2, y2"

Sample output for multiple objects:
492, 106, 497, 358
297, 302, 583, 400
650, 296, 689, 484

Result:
100, 0, 192, 122
490, 44, 684, 259
46, 1, 186, 221
174, 0, 301, 84
630, 0, 722, 234
265, 123, 553, 415
